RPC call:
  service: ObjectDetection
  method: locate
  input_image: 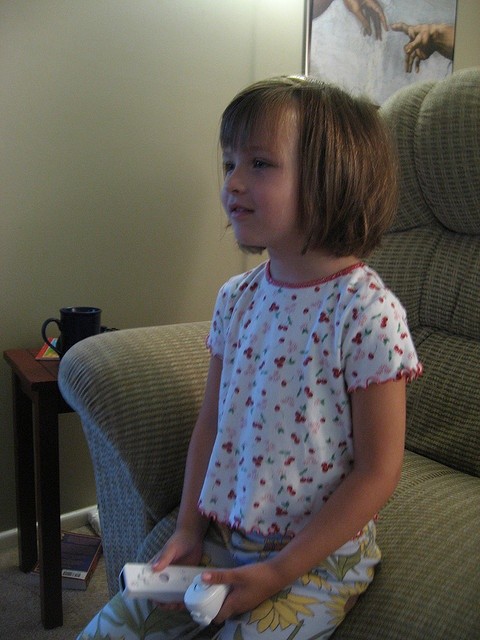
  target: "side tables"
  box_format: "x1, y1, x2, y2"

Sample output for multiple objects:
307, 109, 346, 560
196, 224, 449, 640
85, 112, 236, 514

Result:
5, 347, 77, 631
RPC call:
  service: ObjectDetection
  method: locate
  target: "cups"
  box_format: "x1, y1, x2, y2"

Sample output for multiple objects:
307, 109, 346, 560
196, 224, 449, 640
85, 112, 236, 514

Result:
40, 304, 102, 360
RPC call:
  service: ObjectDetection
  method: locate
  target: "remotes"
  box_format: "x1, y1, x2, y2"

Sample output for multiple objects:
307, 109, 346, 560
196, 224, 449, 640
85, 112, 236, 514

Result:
119, 562, 227, 602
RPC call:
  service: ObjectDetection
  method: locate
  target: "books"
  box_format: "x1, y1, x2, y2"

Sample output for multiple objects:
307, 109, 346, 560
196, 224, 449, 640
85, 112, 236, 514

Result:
31, 532, 103, 592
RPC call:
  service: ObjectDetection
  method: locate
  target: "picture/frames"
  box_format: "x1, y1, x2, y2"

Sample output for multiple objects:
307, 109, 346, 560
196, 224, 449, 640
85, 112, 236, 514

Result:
301, 0, 459, 111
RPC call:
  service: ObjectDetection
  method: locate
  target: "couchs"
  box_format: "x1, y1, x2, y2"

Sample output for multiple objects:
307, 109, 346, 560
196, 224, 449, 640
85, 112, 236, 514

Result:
56, 69, 479, 640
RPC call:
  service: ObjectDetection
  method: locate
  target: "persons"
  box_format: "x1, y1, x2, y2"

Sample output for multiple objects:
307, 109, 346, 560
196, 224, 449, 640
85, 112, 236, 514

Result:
390, 22, 456, 71
78, 76, 422, 640
342, 0, 388, 41
